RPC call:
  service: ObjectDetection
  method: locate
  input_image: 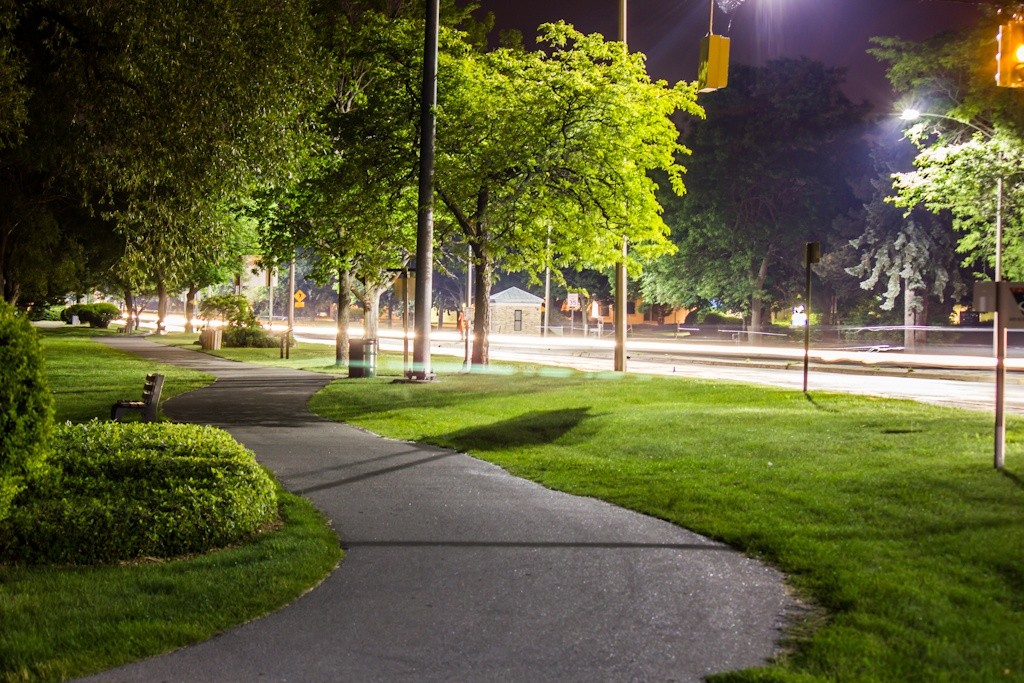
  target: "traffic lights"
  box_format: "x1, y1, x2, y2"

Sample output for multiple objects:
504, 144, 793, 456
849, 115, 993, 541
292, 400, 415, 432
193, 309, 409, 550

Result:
1003, 21, 1024, 87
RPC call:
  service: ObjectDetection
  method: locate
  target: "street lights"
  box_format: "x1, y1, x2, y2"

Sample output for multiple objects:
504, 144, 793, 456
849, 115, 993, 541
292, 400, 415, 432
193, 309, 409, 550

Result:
900, 109, 1010, 358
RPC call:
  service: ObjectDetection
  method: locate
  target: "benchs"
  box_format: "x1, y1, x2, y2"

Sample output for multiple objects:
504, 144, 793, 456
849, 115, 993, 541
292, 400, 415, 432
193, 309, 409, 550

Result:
111, 374, 166, 423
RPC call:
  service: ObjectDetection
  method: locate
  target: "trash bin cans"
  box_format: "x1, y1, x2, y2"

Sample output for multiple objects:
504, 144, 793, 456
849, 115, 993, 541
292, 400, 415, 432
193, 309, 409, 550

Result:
347, 339, 376, 377
